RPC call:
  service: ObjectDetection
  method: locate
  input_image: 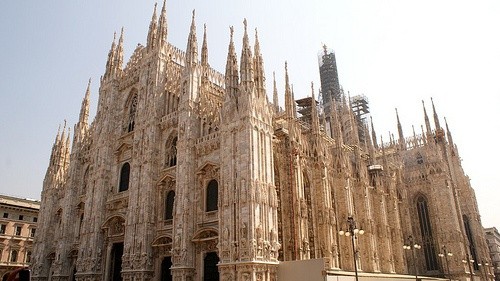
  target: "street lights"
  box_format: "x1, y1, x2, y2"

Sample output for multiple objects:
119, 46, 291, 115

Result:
489, 262, 495, 281
340, 216, 365, 281
403, 235, 423, 280
439, 244, 455, 281
478, 260, 489, 281
462, 254, 476, 281
